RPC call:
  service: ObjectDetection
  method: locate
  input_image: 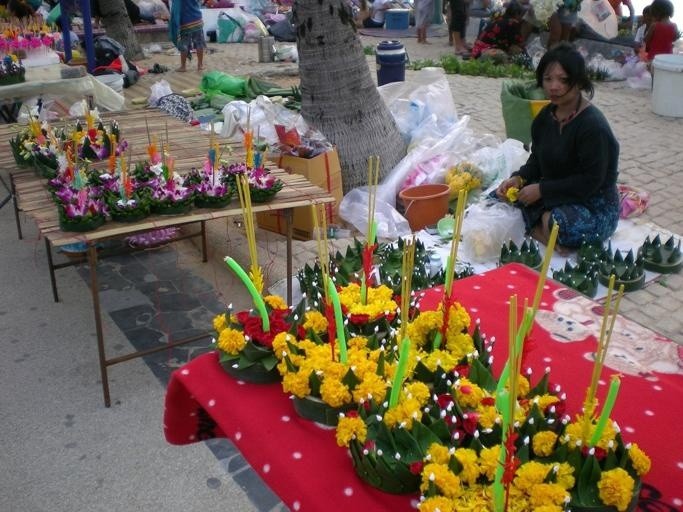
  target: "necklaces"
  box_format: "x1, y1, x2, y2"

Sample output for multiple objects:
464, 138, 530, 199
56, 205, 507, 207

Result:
552, 92, 582, 123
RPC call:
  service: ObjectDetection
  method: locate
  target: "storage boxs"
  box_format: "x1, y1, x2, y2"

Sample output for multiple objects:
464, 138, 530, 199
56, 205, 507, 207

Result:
383, 7, 410, 29
256, 147, 343, 241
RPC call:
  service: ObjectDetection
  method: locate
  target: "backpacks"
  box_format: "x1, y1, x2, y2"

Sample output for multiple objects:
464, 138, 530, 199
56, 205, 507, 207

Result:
81, 35, 140, 88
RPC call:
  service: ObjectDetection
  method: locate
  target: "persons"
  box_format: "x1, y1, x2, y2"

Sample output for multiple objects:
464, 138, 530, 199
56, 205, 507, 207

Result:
634, 0, 681, 69
414, 0, 434, 45
549, 0, 583, 51
170, 0, 208, 72
485, 45, 621, 258
55, 15, 80, 53
470, 0, 535, 61
90, 0, 141, 29
354, 0, 390, 28
606, 0, 635, 19
445, 1, 473, 56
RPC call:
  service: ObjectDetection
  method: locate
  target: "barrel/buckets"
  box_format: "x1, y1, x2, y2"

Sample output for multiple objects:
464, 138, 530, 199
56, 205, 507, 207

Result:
91, 74, 125, 112
258, 35, 274, 62
398, 182, 452, 234
376, 40, 409, 86
649, 54, 683, 118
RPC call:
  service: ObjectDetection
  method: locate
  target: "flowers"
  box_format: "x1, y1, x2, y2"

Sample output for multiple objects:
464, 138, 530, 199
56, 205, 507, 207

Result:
18, 126, 284, 216
125, 227, 177, 244
505, 187, 519, 202
441, 163, 482, 205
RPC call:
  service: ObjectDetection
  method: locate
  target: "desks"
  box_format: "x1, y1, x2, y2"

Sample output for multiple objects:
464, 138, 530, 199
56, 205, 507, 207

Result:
165, 263, 683, 512
0, 108, 336, 407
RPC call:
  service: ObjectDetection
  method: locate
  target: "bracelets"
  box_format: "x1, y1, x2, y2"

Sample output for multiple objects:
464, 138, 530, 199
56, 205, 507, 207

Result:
518, 175, 523, 188
